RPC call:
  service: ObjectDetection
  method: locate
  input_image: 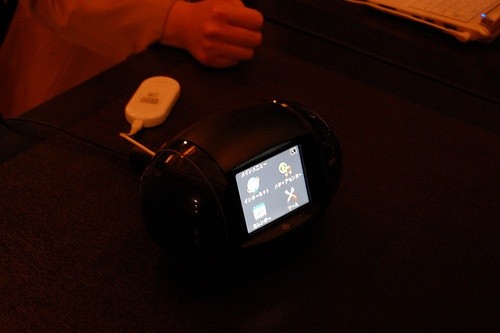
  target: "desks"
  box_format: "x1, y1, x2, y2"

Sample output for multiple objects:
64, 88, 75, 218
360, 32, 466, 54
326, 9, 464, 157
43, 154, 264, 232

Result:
0, 38, 500, 333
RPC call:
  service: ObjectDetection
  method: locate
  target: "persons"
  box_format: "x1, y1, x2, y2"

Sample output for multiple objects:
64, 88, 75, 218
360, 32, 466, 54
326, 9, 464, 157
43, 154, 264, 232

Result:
0, 0, 264, 120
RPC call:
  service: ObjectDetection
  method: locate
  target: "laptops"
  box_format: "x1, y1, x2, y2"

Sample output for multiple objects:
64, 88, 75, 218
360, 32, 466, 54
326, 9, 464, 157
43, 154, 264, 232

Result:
349, 0, 500, 41
